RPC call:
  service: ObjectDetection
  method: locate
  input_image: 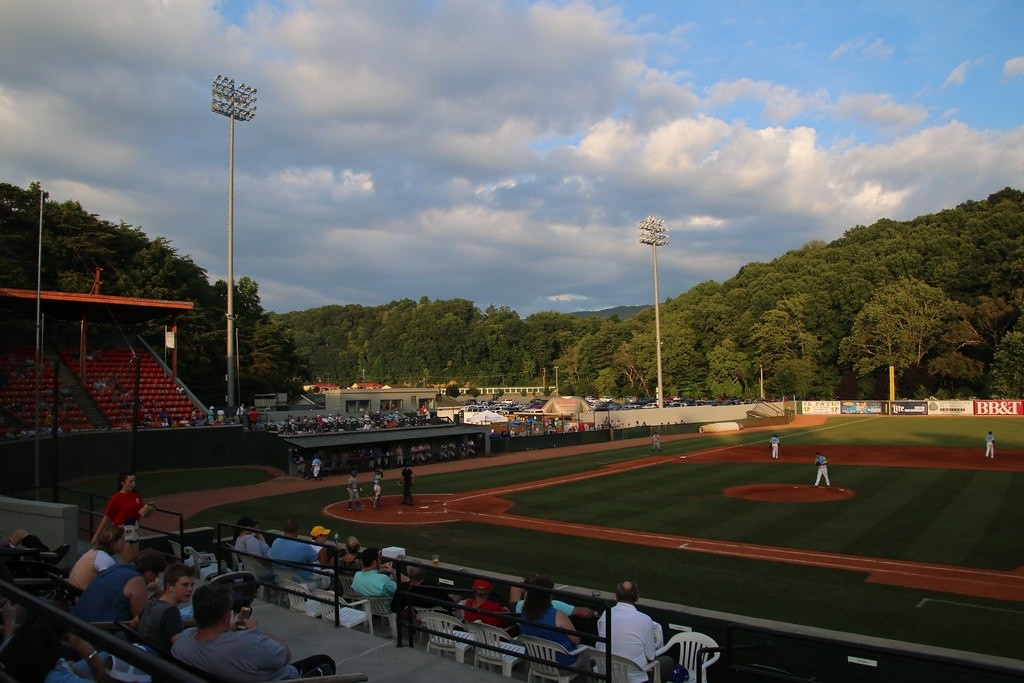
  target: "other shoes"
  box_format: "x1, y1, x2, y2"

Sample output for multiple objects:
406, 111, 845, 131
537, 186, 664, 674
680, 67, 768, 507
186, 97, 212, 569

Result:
357, 508, 363, 510
348, 508, 352, 511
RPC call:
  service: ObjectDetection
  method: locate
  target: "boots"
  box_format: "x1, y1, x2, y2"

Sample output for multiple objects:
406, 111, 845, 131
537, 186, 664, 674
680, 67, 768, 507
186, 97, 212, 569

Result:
22, 535, 71, 565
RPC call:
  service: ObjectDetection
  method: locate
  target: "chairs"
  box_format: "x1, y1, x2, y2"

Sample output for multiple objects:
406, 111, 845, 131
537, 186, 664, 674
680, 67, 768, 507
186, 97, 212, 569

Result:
0, 534, 721, 683
0, 348, 198, 431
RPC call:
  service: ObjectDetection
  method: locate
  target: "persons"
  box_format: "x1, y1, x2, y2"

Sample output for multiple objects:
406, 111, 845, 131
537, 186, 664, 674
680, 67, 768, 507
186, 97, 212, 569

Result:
290, 439, 476, 480
985, 431, 996, 460
277, 404, 431, 435
372, 467, 383, 508
71, 350, 93, 362
0, 523, 336, 683
813, 452, 830, 488
233, 512, 673, 683
90, 471, 152, 564
0, 356, 35, 386
93, 376, 169, 428
507, 417, 685, 436
179, 405, 225, 427
19, 385, 71, 437
347, 469, 363, 510
398, 465, 415, 505
652, 432, 661, 454
175, 384, 184, 395
769, 435, 780, 459
235, 403, 260, 430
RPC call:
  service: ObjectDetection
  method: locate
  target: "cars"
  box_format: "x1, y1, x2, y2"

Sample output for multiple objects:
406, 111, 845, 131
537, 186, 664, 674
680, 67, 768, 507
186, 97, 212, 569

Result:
463, 399, 548, 414
663, 396, 753, 408
585, 395, 659, 412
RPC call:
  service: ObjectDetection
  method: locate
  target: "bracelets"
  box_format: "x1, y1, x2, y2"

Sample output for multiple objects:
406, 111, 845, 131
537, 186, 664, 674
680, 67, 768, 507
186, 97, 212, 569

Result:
87, 650, 97, 661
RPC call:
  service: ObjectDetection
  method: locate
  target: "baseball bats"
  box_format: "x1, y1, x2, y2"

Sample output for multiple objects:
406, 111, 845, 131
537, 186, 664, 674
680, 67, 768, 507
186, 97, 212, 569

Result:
362, 491, 373, 502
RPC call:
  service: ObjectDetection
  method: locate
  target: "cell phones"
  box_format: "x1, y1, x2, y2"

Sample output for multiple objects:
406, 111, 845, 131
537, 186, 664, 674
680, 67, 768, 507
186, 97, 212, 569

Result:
238, 607, 252, 629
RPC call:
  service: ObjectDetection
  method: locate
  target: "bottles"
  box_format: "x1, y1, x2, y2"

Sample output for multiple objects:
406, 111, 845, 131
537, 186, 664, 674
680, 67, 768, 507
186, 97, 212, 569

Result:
334, 531, 339, 543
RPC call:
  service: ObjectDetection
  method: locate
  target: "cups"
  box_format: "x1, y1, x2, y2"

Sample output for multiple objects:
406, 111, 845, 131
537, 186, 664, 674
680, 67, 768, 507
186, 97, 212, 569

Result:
432, 555, 440, 565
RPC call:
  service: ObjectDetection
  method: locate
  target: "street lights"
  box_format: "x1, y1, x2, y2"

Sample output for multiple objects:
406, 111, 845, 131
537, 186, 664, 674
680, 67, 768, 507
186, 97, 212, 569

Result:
554, 365, 559, 396
760, 365, 763, 400
212, 75, 257, 408
638, 216, 669, 408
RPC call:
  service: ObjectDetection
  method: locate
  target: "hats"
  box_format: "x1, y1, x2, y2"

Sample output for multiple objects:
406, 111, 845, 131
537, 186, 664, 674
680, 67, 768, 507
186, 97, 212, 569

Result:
473, 579, 492, 594
376, 469, 380, 472
351, 471, 357, 474
814, 451, 820, 455
310, 526, 331, 536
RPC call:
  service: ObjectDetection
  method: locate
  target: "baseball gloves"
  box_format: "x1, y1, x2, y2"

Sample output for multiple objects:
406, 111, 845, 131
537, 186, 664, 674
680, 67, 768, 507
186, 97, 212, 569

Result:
397, 481, 403, 485
816, 461, 820, 466
